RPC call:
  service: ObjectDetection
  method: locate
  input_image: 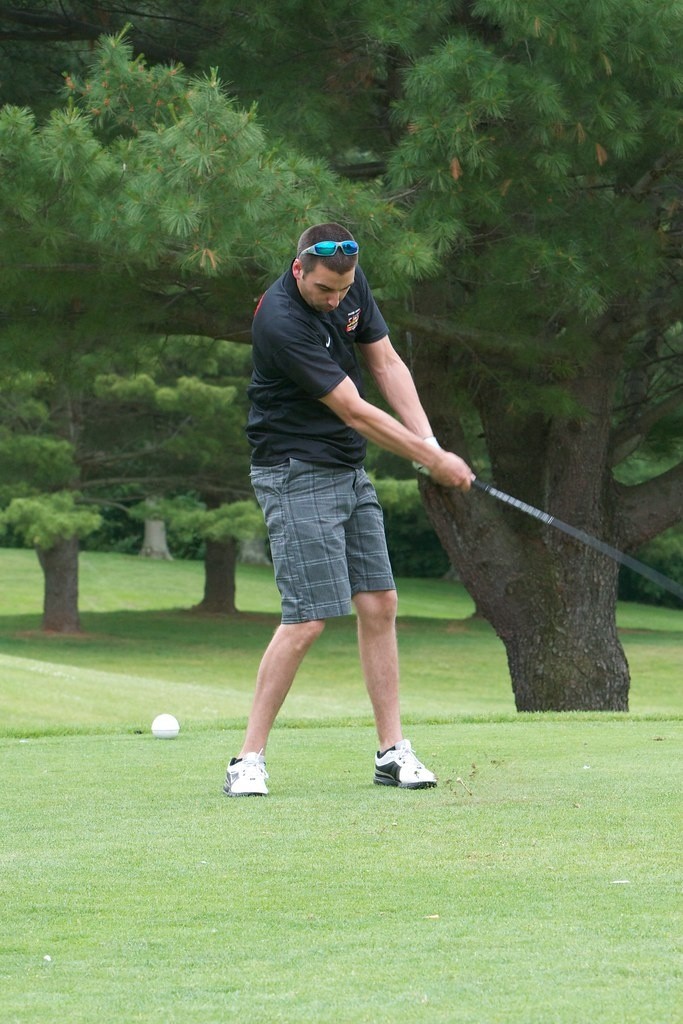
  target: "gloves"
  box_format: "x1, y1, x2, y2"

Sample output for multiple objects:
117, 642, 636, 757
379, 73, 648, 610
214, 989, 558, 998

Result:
412, 434, 443, 477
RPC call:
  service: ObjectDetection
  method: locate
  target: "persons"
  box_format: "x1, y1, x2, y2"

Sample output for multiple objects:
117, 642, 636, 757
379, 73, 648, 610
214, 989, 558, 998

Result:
222, 223, 471, 797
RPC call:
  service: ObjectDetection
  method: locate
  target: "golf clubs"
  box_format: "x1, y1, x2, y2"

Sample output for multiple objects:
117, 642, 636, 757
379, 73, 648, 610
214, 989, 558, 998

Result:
472, 477, 683, 600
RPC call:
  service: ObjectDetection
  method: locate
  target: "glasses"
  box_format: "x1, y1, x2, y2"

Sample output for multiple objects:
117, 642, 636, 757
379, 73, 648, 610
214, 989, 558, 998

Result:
298, 240, 359, 265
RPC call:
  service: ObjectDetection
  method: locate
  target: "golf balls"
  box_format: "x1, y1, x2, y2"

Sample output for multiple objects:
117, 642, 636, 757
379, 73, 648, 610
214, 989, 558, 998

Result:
150, 714, 180, 739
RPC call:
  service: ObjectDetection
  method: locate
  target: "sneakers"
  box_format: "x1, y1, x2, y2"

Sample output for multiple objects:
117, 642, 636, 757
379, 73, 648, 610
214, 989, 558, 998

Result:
373, 738, 438, 789
221, 747, 270, 797
